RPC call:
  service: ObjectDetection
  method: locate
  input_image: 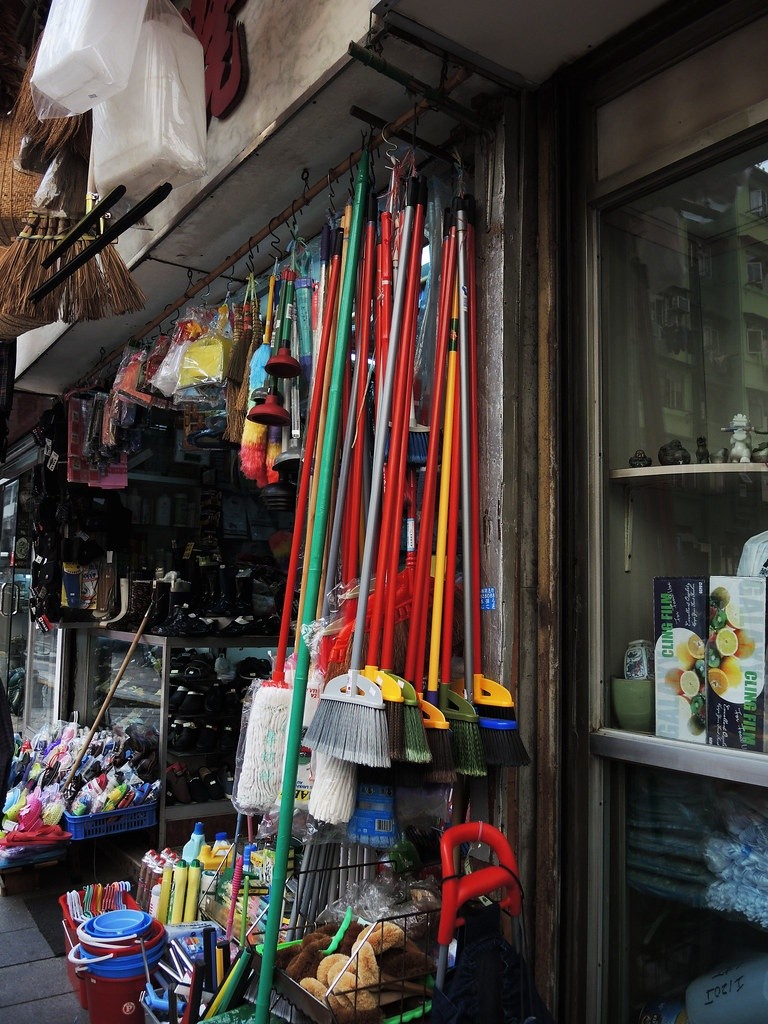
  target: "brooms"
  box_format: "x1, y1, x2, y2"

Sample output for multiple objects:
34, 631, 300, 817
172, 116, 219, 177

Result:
303, 201, 530, 770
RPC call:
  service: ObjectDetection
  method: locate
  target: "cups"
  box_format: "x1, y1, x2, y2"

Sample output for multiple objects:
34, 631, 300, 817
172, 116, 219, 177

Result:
611, 680, 651, 733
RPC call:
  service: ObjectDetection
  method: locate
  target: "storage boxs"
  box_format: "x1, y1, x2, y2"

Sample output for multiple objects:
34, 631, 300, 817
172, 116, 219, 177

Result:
58, 889, 141, 1011
652, 577, 708, 747
60, 801, 158, 840
707, 575, 768, 754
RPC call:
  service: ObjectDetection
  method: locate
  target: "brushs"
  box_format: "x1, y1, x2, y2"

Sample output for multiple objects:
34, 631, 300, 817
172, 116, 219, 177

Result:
273, 906, 435, 1024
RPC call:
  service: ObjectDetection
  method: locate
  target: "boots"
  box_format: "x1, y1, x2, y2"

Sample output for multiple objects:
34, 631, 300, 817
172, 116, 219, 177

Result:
93, 558, 286, 635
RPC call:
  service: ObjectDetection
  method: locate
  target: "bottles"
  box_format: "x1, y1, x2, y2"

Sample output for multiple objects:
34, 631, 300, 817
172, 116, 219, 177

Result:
212, 832, 235, 869
150, 878, 163, 916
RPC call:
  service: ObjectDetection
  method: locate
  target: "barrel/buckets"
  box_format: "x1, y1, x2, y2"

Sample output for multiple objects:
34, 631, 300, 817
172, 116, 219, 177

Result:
69, 912, 167, 1024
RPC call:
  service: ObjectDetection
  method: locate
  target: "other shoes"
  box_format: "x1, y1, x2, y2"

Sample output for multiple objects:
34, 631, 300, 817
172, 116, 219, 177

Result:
92, 647, 274, 804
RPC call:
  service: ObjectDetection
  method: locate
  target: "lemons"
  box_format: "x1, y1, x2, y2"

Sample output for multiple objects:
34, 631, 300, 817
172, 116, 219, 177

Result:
666, 587, 755, 737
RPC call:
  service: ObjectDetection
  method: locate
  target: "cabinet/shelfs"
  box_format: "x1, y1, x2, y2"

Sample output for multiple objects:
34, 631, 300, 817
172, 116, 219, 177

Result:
572, 99, 768, 1024
84, 628, 295, 854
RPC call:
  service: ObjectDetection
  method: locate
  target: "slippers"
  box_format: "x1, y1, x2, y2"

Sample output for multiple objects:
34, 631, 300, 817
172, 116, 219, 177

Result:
0, 724, 160, 857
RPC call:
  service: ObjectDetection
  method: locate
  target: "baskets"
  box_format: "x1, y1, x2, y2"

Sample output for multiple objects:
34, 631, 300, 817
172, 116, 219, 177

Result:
62, 800, 159, 840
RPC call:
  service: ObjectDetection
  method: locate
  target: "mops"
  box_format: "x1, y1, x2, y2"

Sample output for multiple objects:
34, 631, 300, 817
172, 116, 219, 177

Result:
237, 153, 461, 839
